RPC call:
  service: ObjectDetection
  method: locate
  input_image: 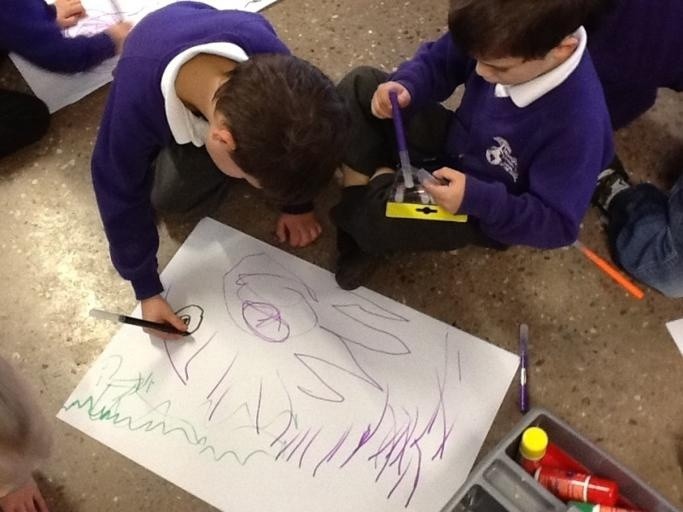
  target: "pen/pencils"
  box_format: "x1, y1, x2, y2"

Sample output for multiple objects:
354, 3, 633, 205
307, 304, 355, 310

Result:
387, 85, 417, 193
573, 238, 646, 303
518, 323, 530, 415
86, 305, 193, 336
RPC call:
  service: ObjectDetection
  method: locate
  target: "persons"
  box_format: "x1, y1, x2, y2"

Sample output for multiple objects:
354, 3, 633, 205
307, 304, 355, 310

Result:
1, 0, 133, 162
91, 0, 354, 342
590, 151, 683, 299
0, 358, 54, 512
328, 0, 615, 291
586, 0, 683, 130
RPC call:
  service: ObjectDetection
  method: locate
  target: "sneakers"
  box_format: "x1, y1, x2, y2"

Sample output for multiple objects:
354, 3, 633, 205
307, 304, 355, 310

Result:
335, 224, 383, 290
